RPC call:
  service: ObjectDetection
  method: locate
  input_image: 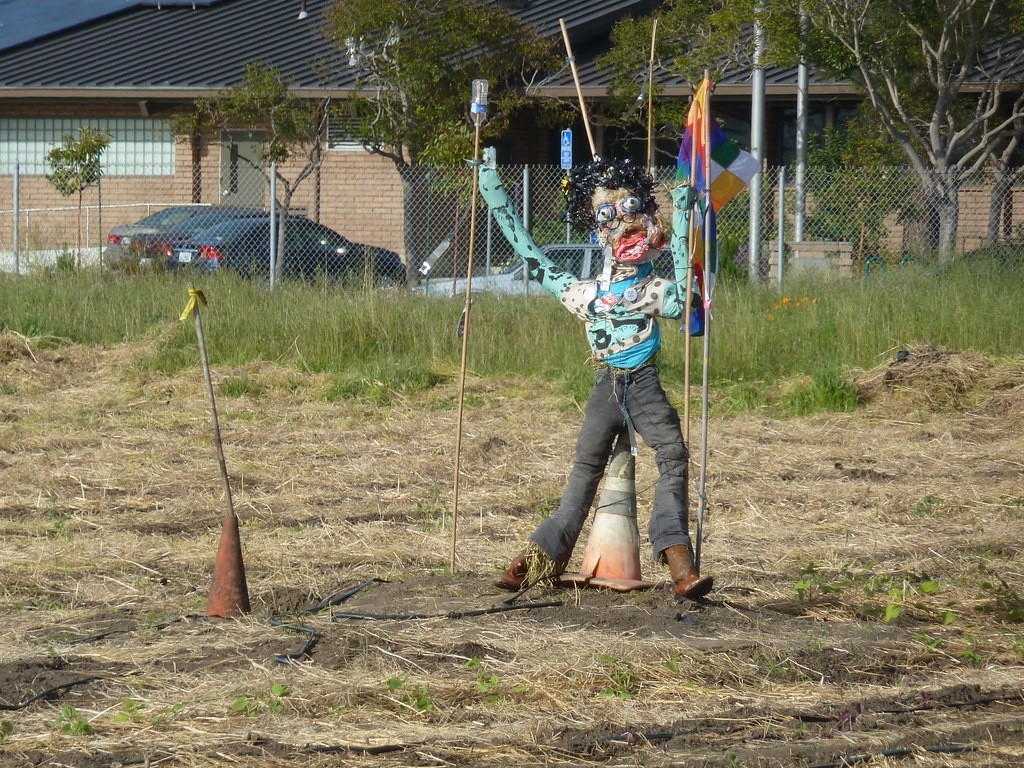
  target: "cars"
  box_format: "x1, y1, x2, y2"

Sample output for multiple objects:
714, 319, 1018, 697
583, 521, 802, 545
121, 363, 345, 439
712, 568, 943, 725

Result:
157, 216, 407, 297
410, 244, 675, 304
102, 206, 271, 276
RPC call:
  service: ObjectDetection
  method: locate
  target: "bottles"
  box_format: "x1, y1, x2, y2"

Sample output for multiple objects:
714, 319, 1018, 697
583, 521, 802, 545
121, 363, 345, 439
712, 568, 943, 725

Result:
470, 79, 488, 127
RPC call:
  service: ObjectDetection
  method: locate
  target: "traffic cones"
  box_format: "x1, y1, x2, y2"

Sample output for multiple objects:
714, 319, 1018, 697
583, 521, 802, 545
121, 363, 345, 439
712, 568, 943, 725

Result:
558, 416, 660, 592
200, 514, 255, 621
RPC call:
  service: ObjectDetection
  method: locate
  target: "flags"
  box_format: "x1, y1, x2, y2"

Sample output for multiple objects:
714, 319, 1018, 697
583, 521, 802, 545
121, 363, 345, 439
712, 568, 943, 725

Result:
675, 79, 760, 337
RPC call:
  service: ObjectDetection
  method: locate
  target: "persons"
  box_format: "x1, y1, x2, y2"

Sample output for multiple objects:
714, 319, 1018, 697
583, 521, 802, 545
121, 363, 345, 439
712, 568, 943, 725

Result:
478, 146, 712, 605
